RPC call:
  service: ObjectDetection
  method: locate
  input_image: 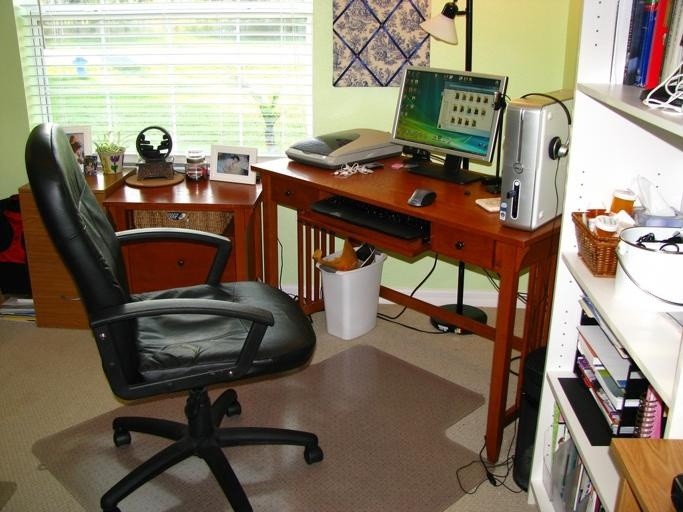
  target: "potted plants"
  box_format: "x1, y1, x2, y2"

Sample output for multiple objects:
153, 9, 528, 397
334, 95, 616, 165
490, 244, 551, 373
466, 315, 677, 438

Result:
93, 129, 126, 176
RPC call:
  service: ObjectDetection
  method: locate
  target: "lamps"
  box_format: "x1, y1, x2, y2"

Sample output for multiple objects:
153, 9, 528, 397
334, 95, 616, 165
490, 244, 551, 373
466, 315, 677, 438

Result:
417, 0, 468, 46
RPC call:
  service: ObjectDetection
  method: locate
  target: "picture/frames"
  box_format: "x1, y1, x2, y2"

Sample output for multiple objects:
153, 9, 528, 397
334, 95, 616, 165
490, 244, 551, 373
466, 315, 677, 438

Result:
207, 144, 258, 187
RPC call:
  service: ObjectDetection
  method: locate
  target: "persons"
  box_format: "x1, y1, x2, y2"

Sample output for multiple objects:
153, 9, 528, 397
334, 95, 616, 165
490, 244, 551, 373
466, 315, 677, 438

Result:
84, 160, 94, 176
223, 155, 241, 175
68, 134, 81, 161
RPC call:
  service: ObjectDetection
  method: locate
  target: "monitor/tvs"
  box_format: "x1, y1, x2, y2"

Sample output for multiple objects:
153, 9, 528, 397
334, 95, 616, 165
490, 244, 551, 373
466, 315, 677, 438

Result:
389, 66, 509, 184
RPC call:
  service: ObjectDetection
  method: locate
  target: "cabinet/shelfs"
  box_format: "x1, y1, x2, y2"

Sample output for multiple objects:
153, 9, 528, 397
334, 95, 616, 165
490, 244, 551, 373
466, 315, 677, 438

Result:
524, 2, 682, 511
101, 178, 265, 293
16, 164, 138, 330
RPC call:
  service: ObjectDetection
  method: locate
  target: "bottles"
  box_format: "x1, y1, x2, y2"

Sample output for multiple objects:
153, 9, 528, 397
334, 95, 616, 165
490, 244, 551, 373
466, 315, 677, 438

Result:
184, 156, 206, 183
608, 189, 635, 218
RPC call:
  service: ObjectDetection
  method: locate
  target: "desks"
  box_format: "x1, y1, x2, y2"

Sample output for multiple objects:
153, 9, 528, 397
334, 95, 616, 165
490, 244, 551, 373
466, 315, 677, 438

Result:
249, 155, 563, 464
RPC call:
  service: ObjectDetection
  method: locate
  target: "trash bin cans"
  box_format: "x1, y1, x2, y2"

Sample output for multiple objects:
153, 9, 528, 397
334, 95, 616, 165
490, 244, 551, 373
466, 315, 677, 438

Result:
315, 247, 387, 341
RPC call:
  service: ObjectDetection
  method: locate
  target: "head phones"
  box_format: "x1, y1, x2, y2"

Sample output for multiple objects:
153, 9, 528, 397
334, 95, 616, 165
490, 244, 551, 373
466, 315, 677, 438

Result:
520, 93, 571, 160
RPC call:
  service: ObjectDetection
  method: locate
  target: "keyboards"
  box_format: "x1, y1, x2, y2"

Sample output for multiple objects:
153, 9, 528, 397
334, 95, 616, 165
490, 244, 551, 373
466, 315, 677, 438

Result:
311, 195, 430, 240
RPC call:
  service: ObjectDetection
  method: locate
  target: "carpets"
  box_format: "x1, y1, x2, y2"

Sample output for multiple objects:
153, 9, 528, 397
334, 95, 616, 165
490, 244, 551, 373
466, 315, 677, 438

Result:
28, 342, 500, 512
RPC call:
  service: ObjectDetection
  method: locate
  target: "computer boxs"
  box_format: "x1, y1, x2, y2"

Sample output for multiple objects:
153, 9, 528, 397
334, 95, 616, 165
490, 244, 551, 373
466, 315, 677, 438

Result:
499, 89, 573, 233
513, 346, 547, 492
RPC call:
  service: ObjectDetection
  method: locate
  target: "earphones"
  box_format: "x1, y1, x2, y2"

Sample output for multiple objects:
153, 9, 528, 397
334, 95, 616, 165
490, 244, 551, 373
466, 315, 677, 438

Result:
334, 168, 341, 175
340, 171, 348, 176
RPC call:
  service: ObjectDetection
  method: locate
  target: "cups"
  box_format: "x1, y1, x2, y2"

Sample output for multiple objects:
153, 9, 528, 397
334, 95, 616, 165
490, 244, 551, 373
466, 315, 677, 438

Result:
594, 214, 619, 238
587, 208, 606, 223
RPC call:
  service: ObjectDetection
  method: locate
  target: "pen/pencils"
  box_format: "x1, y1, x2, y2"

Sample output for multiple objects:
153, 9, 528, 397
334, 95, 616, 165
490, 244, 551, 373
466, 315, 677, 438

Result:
391, 164, 418, 169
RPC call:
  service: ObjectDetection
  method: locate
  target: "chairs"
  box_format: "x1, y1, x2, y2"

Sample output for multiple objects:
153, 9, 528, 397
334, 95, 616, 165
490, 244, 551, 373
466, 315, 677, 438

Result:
19, 117, 324, 511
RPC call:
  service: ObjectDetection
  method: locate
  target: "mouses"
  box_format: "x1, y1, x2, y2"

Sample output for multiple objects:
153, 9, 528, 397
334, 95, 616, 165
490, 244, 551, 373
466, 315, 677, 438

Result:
407, 188, 437, 207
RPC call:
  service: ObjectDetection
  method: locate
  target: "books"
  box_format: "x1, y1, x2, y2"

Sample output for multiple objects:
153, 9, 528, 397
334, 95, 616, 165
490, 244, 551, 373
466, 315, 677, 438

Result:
623, 1, 683, 91
0, 293, 35, 324
543, 295, 665, 512
